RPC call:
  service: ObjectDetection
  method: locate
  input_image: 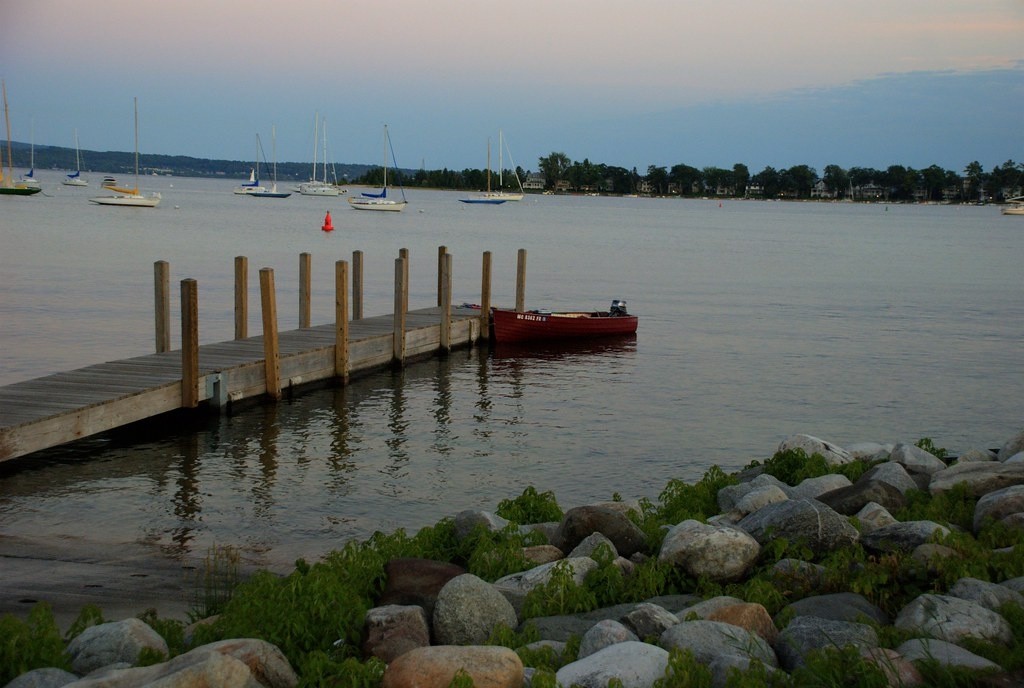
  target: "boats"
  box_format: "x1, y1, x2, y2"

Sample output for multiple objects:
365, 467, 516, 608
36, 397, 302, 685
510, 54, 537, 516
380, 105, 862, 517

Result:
100, 175, 116, 188
491, 299, 639, 343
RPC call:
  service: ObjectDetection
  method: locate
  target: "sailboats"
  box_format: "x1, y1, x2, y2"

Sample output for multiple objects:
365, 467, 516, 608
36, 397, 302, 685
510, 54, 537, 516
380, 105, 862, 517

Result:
482, 130, 525, 201
361, 124, 388, 198
0, 78, 42, 195
12, 118, 38, 184
293, 112, 348, 197
88, 96, 162, 207
62, 128, 89, 186
1002, 168, 1024, 215
457, 140, 506, 204
252, 126, 292, 198
232, 133, 272, 195
349, 130, 408, 211
